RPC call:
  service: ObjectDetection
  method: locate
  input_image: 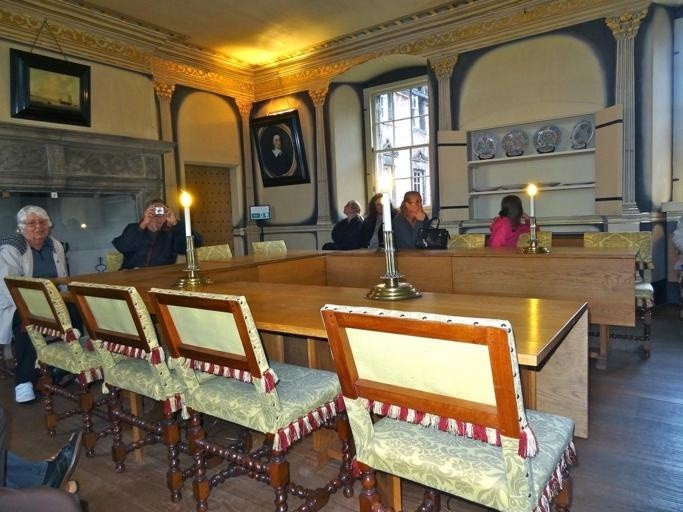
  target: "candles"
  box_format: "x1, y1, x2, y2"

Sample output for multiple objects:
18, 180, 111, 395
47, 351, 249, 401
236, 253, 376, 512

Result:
527, 181, 537, 216
179, 192, 192, 236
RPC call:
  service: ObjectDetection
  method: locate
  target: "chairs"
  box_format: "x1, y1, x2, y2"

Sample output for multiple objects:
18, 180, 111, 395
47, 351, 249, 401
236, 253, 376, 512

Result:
515, 230, 552, 249
147, 285, 356, 512
447, 233, 485, 248
318, 300, 577, 512
581, 230, 653, 359
380, 173, 392, 231
71, 281, 247, 502
3, 274, 125, 457
251, 238, 287, 254
194, 243, 233, 261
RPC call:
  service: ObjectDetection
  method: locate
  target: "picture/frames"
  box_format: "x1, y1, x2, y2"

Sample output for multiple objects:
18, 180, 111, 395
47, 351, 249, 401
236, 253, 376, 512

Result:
8, 44, 92, 128
250, 107, 311, 188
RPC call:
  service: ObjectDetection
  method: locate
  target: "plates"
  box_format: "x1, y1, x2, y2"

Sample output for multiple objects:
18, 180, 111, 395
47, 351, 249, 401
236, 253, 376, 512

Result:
533, 126, 560, 153
472, 134, 497, 157
570, 119, 594, 147
501, 129, 529, 154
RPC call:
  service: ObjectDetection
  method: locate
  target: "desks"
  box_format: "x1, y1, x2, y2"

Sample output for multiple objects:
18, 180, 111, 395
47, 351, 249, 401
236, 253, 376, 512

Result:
325, 247, 635, 369
54, 250, 326, 285
47, 278, 591, 511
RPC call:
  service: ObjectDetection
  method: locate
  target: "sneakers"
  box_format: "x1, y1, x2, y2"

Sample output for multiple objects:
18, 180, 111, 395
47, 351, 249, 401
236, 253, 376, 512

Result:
43, 432, 83, 489
15, 382, 35, 403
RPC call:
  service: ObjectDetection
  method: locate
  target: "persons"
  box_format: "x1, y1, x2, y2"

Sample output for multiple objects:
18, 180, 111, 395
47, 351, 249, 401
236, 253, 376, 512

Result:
322, 199, 365, 250
485, 194, 540, 249
360, 192, 397, 249
0, 204, 70, 405
0, 485, 82, 512
0, 412, 85, 489
391, 190, 430, 250
268, 132, 291, 177
109, 198, 204, 271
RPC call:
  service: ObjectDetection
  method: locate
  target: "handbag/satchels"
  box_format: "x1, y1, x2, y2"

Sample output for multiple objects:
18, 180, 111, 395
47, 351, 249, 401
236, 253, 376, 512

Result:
416, 228, 448, 248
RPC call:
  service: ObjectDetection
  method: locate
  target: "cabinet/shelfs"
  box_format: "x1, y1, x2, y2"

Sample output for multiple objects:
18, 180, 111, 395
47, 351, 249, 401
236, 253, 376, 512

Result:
465, 111, 605, 216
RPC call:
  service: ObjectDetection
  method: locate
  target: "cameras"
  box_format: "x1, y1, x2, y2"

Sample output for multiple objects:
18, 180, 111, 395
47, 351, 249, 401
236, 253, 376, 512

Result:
153, 207, 164, 215
